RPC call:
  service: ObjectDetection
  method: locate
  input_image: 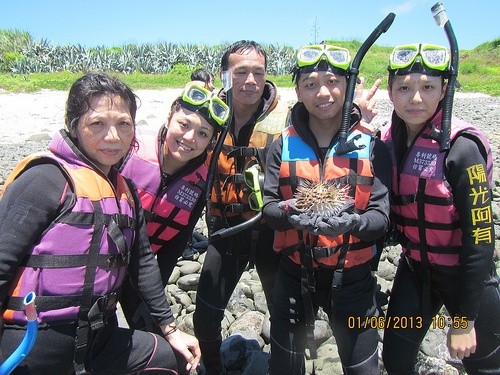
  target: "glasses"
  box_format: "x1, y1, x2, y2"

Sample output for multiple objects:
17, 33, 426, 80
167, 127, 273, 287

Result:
244, 169, 263, 211
295, 45, 351, 71
389, 44, 449, 71
183, 84, 231, 126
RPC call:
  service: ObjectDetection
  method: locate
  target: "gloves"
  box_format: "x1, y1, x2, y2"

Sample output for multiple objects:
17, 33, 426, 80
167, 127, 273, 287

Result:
311, 211, 360, 237
277, 198, 330, 229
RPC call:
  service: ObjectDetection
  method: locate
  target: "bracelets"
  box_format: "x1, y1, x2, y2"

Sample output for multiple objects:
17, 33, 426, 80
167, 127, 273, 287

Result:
164, 324, 179, 337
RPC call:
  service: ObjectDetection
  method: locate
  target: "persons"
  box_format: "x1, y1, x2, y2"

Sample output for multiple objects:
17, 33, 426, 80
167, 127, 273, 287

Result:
380, 42, 500, 375
191, 67, 216, 92
0, 72, 201, 375
191, 41, 379, 375
106, 78, 231, 339
262, 43, 390, 374
